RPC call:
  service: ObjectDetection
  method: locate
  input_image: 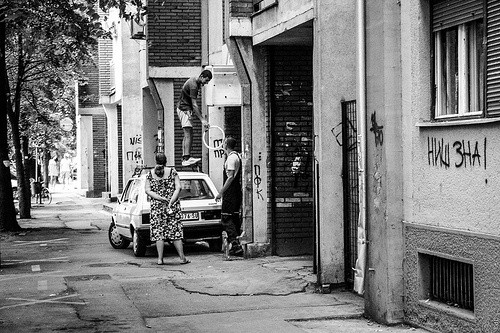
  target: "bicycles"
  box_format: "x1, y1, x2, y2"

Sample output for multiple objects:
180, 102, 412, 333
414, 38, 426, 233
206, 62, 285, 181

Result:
34, 181, 52, 205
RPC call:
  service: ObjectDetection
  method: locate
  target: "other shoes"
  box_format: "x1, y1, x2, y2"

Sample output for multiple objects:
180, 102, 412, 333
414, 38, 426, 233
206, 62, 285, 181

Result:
229, 246, 242, 254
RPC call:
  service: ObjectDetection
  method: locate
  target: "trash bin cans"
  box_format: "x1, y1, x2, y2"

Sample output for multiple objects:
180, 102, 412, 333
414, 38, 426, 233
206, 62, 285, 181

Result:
31, 181, 40, 194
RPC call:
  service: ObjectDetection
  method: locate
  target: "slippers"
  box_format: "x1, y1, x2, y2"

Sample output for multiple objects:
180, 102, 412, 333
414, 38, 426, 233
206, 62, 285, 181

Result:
157, 261, 164, 265
180, 261, 191, 264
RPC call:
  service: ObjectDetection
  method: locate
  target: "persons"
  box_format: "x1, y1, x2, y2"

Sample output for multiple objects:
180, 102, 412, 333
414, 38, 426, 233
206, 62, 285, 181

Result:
48, 154, 58, 187
213, 136, 246, 256
176, 69, 213, 166
145, 152, 192, 264
60, 153, 71, 190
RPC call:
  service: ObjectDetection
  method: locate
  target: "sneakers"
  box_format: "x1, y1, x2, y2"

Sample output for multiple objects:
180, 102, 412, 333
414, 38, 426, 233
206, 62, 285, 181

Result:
181, 157, 201, 167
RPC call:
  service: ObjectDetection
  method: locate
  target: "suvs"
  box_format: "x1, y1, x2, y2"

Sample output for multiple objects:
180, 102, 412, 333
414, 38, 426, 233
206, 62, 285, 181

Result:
108, 164, 224, 257
